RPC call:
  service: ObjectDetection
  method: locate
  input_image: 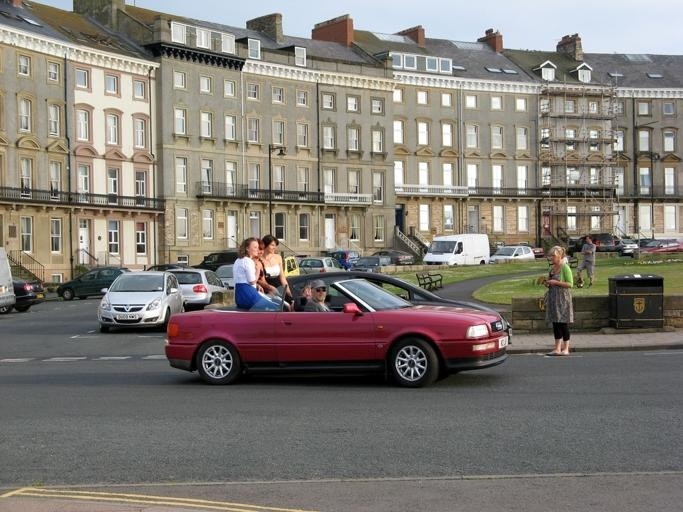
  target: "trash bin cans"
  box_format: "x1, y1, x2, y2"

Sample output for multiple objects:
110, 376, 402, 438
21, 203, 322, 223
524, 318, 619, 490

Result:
606, 273, 665, 329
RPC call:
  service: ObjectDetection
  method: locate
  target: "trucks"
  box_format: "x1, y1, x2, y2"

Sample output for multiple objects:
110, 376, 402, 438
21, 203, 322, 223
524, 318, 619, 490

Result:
421, 233, 490, 267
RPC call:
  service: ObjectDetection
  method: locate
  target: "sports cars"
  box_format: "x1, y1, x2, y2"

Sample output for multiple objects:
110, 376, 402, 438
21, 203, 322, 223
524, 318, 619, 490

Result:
163, 274, 510, 386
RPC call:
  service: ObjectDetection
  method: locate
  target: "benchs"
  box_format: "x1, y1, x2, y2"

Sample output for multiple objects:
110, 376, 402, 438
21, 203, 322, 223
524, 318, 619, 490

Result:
547, 255, 578, 268
415, 271, 442, 290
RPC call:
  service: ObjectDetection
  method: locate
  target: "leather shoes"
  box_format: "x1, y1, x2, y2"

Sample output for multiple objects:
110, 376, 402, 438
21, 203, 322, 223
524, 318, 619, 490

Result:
546, 350, 560, 355
557, 351, 570, 356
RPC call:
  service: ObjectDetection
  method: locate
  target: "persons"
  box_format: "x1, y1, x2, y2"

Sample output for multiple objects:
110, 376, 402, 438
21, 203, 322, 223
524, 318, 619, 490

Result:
576, 235, 596, 287
543, 246, 575, 356
305, 280, 333, 313
338, 253, 351, 270
262, 235, 294, 307
233, 237, 279, 311
301, 286, 312, 300
254, 240, 291, 311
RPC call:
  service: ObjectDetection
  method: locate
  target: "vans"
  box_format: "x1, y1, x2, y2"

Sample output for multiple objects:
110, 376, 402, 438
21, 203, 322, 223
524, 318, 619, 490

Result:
329, 250, 361, 272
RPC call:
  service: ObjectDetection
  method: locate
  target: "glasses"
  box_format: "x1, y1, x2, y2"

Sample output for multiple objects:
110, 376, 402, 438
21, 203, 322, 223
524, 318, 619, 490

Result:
316, 288, 326, 292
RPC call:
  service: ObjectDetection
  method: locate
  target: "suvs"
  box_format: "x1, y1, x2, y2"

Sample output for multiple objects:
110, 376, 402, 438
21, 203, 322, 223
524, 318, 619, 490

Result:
199, 249, 240, 271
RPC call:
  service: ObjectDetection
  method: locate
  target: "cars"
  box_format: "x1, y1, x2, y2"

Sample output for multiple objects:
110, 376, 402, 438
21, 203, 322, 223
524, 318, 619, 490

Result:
369, 250, 415, 266
616, 238, 642, 259
97, 271, 186, 333
163, 268, 228, 311
634, 238, 683, 255
487, 245, 535, 265
56, 266, 132, 301
504, 243, 544, 258
569, 234, 615, 258
213, 264, 233, 288
350, 256, 382, 275
297, 256, 348, 276
633, 238, 654, 248
145, 264, 191, 271
10, 265, 46, 313
282, 256, 300, 277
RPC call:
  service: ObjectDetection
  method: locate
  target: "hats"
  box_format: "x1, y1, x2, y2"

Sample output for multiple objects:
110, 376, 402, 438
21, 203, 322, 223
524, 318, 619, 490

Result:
310, 278, 327, 289
